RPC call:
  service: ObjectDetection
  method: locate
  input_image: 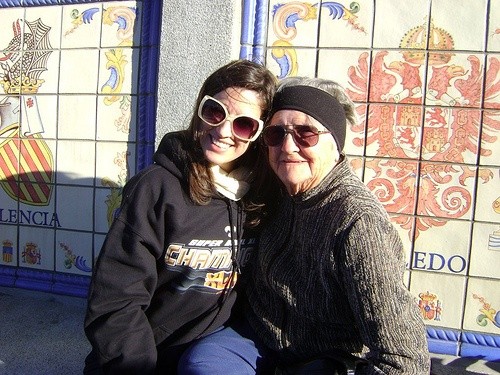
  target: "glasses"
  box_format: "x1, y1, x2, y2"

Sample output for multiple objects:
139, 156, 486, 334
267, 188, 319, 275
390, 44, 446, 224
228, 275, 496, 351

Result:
261, 123, 342, 149
197, 94, 263, 145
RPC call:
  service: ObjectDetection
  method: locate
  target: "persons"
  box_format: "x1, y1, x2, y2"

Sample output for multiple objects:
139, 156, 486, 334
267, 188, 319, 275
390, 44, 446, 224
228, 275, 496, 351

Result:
239, 75, 432, 375
83, 57, 276, 375
